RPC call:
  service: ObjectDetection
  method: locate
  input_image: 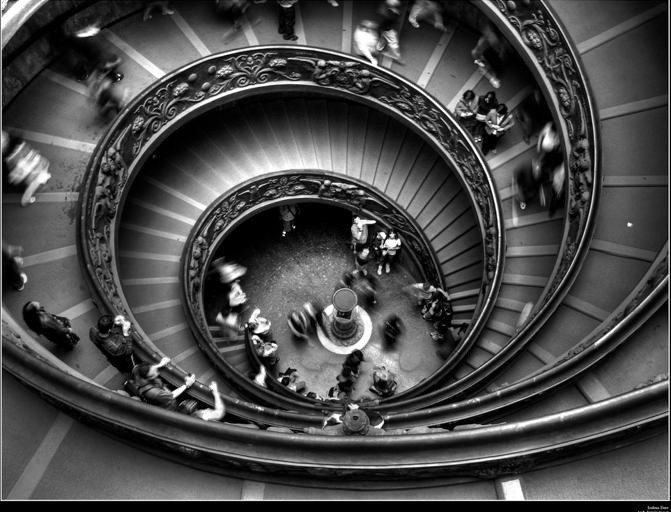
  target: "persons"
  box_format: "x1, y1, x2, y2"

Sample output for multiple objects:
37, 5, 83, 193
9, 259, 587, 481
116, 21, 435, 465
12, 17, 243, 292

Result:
2, 0, 564, 434
